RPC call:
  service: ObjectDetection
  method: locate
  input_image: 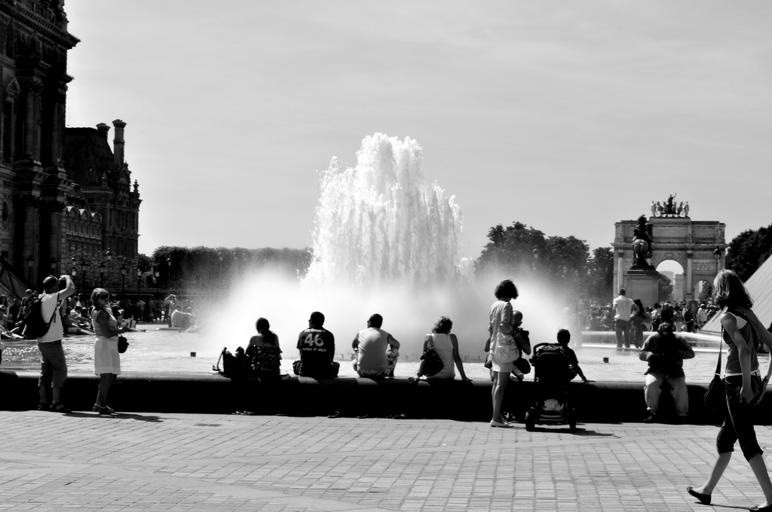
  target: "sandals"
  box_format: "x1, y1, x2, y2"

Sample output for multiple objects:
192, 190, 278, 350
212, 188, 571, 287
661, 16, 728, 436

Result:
49, 401, 71, 413
36, 402, 47, 410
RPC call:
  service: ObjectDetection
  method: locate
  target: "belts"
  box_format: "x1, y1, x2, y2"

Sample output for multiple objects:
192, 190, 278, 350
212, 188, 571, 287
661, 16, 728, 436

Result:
722, 368, 761, 378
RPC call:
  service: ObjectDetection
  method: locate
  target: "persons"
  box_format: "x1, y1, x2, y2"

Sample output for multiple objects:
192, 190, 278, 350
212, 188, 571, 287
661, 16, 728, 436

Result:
246, 318, 282, 381
92, 288, 128, 414
610, 288, 640, 353
417, 316, 472, 383
631, 299, 647, 349
485, 311, 531, 381
351, 314, 400, 379
639, 322, 694, 422
648, 291, 706, 323
292, 312, 340, 379
529, 329, 595, 383
483, 280, 520, 428
1, 289, 29, 342
687, 269, 772, 511
37, 275, 75, 412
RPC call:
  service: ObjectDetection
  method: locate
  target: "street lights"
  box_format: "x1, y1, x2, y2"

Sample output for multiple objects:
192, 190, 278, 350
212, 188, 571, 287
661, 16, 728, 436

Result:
120, 265, 127, 301
98, 263, 106, 288
137, 268, 142, 295
51, 261, 57, 276
82, 263, 88, 286
155, 271, 160, 303
28, 259, 35, 290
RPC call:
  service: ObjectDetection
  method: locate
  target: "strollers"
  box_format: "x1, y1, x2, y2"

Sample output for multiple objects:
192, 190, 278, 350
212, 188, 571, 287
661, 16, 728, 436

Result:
525, 343, 575, 430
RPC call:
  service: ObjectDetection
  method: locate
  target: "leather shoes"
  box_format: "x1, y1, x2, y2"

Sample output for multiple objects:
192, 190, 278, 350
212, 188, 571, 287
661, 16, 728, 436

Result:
686, 486, 711, 507
490, 419, 513, 428
750, 501, 769, 510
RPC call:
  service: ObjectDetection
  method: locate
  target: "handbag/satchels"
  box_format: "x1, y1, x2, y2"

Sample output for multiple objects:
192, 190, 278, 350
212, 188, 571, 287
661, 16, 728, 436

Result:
118, 331, 128, 353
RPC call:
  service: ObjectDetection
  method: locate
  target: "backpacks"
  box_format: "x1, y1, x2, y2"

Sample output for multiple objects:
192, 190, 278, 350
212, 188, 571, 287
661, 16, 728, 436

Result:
19, 292, 61, 340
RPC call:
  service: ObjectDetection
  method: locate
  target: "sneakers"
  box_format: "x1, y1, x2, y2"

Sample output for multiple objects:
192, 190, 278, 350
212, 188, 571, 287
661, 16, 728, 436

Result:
96, 406, 112, 415
93, 402, 114, 413
